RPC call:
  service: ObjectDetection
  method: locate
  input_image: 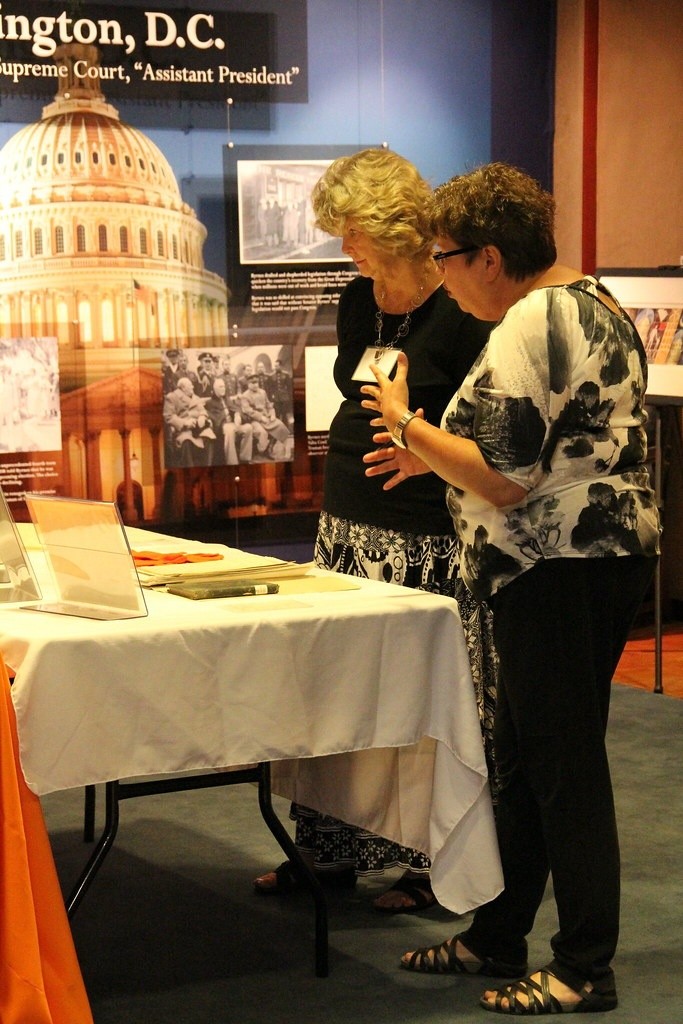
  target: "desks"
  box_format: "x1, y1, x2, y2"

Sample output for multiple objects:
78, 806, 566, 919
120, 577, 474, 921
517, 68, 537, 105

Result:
0, 522, 505, 980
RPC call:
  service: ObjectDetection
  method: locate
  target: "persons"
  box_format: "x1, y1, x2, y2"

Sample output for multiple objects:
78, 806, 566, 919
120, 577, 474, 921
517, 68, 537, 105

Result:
163, 349, 293, 467
360, 163, 661, 1017
0, 366, 59, 427
251, 149, 498, 915
258, 198, 316, 249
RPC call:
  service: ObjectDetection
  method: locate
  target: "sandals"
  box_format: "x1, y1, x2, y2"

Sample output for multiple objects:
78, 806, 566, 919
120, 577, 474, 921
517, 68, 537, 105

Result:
479, 964, 616, 1016
372, 875, 439, 914
253, 854, 356, 894
400, 929, 526, 979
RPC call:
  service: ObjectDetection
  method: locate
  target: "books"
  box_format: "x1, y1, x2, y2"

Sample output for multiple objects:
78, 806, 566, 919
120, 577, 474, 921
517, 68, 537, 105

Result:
166, 580, 279, 600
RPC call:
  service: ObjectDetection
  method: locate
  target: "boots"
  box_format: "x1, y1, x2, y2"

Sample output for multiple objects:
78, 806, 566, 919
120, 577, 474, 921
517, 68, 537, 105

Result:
264, 437, 276, 460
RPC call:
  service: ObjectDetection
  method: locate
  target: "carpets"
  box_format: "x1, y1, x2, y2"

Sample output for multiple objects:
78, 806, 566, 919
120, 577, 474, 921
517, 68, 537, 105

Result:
611, 617, 683, 702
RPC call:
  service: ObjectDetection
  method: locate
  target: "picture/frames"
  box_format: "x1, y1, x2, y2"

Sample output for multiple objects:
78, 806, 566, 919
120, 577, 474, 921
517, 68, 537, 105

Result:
221, 143, 390, 327
596, 267, 683, 405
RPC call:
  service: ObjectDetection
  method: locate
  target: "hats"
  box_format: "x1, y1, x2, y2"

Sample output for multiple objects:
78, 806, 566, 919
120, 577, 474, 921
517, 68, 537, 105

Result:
166, 350, 179, 357
247, 375, 260, 383
199, 353, 212, 360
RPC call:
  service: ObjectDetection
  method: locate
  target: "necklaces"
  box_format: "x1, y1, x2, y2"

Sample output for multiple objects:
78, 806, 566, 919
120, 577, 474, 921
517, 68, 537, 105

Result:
375, 259, 430, 364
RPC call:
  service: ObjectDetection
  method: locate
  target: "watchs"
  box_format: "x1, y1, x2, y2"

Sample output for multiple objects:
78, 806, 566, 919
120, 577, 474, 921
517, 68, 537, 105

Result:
391, 412, 418, 450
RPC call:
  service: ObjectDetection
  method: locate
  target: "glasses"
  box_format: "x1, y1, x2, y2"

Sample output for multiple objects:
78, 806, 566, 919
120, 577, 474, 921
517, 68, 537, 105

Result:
433, 243, 504, 274
